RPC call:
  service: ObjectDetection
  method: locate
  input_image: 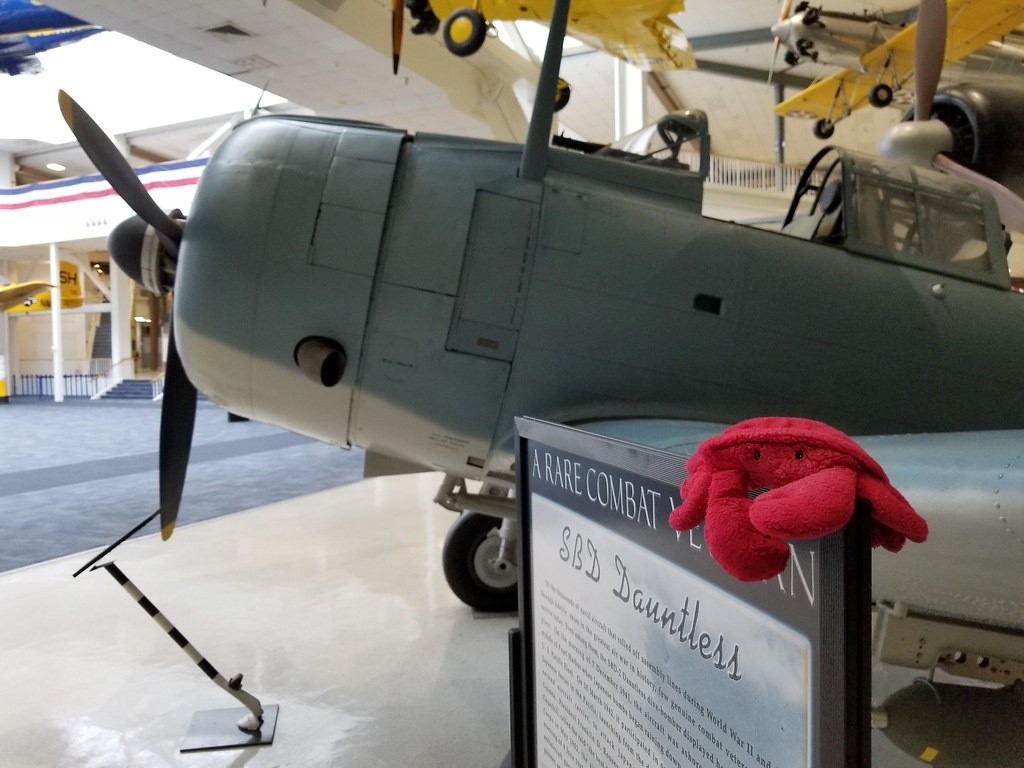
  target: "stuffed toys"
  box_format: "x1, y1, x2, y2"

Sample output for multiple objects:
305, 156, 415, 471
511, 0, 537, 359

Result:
670, 418, 928, 582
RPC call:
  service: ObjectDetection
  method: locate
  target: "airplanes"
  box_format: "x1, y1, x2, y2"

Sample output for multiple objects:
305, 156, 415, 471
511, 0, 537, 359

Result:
0, 0, 1024, 753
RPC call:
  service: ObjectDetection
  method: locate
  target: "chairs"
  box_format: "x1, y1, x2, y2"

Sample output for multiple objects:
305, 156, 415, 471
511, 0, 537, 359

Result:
778, 179, 842, 241
